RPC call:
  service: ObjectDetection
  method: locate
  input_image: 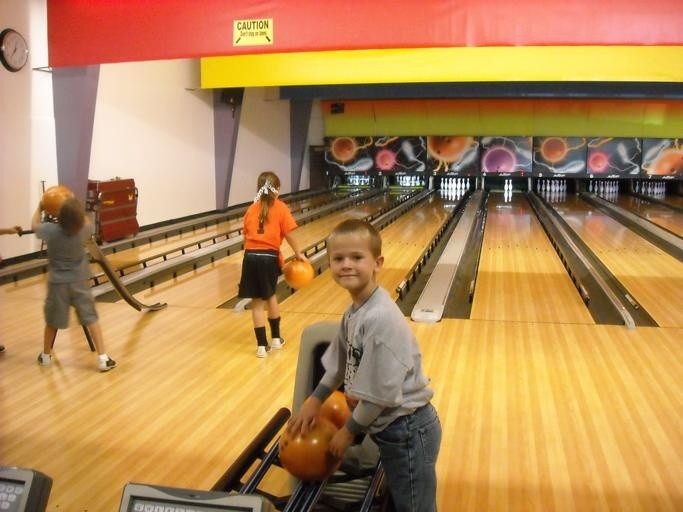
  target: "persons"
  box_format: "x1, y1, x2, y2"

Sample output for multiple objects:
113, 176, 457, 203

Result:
30, 196, 117, 371
285, 218, 442, 512
236, 171, 307, 358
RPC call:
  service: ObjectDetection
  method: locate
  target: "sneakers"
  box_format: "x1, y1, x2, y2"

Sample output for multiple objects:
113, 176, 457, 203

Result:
270, 337, 286, 350
256, 345, 273, 357
98, 357, 119, 371
38, 352, 53, 365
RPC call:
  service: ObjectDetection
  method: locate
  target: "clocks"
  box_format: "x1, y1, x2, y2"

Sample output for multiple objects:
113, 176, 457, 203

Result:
0, 29, 28, 73
